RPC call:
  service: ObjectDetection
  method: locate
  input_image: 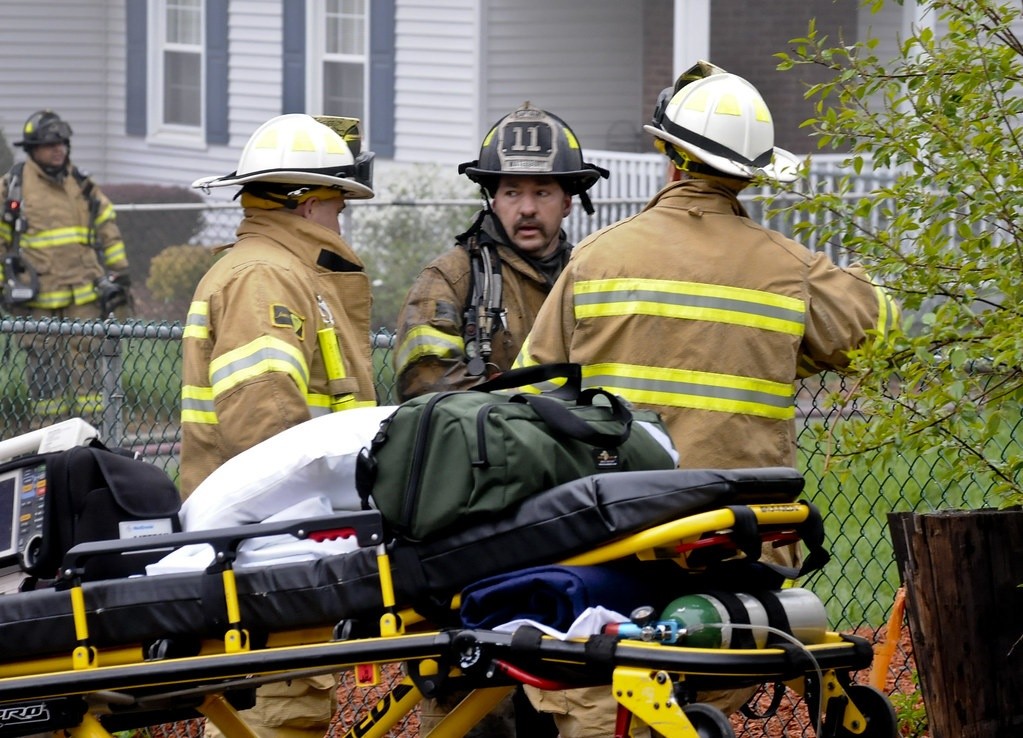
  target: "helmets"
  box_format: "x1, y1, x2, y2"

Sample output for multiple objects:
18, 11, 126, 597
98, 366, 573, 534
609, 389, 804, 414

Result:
644, 59, 804, 183
12, 109, 69, 146
458, 99, 600, 197
193, 111, 375, 198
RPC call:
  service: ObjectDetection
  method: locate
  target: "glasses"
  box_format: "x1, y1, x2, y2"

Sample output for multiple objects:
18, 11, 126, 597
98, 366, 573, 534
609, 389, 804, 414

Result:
652, 85, 674, 122
38, 119, 72, 142
348, 148, 373, 187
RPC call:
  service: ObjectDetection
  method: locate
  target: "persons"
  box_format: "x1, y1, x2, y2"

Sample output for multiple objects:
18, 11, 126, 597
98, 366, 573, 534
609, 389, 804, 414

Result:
0, 112, 132, 433
177, 114, 379, 738
510, 60, 904, 738
392, 101, 609, 404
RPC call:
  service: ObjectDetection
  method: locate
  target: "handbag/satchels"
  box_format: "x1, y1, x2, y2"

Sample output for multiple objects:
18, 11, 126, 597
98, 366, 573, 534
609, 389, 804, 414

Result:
29, 441, 183, 582
355, 356, 681, 542
